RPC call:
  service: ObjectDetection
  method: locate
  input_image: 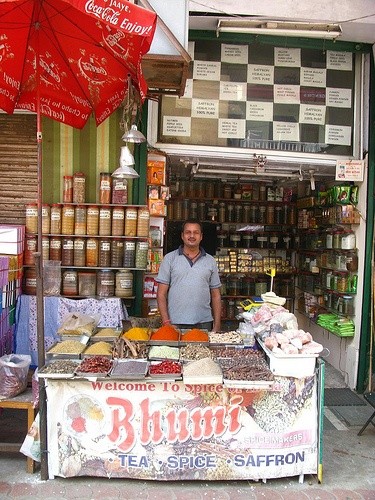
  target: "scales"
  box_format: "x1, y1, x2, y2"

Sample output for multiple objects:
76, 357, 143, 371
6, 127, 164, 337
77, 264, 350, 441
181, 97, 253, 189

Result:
240, 267, 276, 311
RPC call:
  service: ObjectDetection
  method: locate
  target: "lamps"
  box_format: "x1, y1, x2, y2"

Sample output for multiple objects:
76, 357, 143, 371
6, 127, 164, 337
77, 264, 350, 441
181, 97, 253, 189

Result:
216, 19, 343, 41
180, 159, 303, 178
112, 125, 147, 179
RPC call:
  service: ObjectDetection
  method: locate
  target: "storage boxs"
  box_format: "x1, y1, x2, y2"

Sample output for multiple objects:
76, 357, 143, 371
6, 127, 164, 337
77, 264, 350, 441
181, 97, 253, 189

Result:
0, 225, 25, 358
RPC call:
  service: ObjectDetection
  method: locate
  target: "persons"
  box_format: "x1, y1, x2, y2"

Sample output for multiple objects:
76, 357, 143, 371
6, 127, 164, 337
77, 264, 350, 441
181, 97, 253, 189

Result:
154, 219, 222, 332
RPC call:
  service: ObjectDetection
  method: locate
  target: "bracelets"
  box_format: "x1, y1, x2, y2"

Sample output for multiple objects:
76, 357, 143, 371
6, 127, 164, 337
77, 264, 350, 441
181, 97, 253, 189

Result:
162, 320, 171, 324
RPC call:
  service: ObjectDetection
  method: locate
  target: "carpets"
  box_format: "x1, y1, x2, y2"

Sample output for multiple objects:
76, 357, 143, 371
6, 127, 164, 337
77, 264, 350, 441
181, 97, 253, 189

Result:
323, 388, 366, 406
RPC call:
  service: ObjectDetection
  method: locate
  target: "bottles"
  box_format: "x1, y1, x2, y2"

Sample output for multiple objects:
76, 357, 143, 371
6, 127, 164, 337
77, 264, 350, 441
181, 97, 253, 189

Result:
24, 172, 149, 297
166, 180, 293, 319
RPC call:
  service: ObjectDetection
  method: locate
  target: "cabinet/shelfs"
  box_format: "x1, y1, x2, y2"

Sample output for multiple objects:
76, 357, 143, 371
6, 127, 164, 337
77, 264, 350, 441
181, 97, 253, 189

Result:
23, 173, 358, 374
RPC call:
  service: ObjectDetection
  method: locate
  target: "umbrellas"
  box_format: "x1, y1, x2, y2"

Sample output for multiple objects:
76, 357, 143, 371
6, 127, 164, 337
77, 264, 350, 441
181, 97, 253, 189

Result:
0, 0, 160, 480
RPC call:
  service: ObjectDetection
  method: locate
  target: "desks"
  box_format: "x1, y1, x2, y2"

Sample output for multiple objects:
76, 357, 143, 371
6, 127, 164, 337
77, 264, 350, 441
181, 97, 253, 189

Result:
18, 357, 325, 484
0, 388, 41, 474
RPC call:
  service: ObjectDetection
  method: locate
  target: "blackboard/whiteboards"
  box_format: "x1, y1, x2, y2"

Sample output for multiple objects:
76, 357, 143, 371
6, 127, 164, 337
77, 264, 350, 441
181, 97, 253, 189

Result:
165, 220, 223, 256
